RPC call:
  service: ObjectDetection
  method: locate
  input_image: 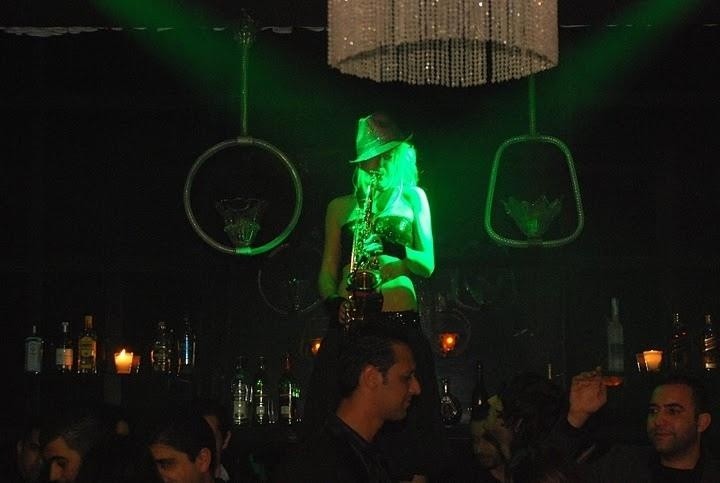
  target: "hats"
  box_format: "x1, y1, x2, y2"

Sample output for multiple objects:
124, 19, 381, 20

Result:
348, 111, 415, 163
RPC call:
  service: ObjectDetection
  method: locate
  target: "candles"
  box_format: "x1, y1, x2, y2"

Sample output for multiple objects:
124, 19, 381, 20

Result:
643, 350, 663, 372
114, 349, 133, 374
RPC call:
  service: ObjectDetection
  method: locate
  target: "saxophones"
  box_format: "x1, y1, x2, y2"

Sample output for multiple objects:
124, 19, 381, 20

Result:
342, 170, 384, 332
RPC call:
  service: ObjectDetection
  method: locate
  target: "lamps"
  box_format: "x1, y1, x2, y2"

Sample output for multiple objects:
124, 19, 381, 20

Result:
327, 0, 559, 88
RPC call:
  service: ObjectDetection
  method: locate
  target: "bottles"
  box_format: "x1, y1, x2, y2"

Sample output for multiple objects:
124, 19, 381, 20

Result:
180, 312, 201, 369
77, 315, 97, 375
230, 349, 302, 428
472, 363, 488, 419
23, 324, 45, 376
149, 321, 183, 377
56, 322, 74, 373
701, 313, 718, 376
438, 375, 462, 426
668, 312, 691, 373
606, 297, 625, 376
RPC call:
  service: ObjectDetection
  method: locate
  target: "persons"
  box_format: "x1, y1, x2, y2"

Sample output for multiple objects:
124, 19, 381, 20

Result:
459, 365, 719, 483
303, 110, 443, 444
284, 324, 427, 483
1, 400, 253, 483
